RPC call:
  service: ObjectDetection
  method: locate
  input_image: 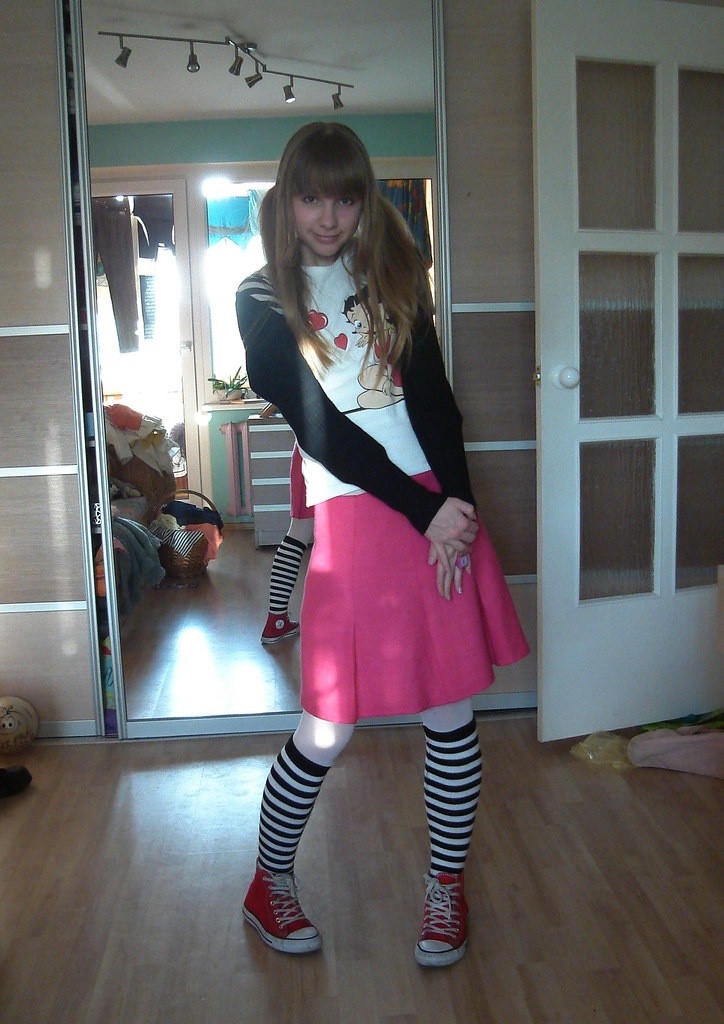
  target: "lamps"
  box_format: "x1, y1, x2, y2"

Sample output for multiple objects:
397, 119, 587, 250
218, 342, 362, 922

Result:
99, 28, 357, 109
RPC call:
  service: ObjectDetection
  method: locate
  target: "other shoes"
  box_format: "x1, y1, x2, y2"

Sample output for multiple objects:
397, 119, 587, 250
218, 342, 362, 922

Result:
0, 764, 32, 797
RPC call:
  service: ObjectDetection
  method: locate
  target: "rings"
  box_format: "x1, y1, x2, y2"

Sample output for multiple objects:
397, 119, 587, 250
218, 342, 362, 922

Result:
456, 554, 468, 568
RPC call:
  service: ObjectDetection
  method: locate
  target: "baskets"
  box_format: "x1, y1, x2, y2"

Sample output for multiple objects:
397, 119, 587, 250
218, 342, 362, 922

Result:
151, 489, 218, 577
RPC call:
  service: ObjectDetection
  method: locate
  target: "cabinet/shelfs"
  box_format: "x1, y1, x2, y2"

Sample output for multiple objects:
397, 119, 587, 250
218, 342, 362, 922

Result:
198, 403, 270, 529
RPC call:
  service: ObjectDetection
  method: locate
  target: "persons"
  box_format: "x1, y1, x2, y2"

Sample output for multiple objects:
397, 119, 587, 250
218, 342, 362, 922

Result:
234, 120, 531, 967
258, 438, 316, 645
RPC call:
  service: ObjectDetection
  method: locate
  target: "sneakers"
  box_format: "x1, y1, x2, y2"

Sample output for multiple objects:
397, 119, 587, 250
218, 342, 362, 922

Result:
242, 859, 322, 954
414, 868, 468, 967
261, 611, 300, 644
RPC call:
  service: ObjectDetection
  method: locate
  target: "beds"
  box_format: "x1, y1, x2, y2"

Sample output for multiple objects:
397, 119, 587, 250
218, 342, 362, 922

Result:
111, 495, 151, 527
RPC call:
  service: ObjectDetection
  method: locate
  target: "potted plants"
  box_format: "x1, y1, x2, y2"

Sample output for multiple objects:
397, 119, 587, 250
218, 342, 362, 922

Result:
206, 365, 248, 402
